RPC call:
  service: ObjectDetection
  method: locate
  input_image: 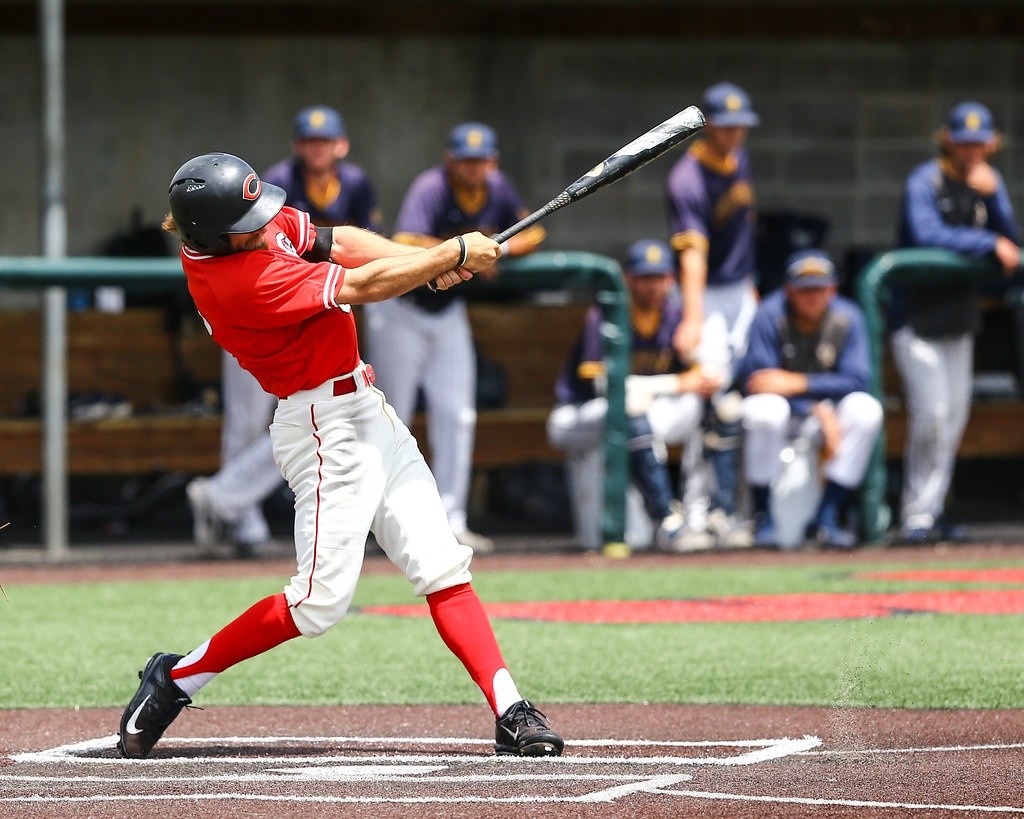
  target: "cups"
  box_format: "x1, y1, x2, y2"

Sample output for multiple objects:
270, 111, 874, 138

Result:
96, 286, 124, 312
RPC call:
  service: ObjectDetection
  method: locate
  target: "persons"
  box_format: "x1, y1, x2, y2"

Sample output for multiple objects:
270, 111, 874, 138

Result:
365, 122, 544, 555
881, 103, 1024, 541
548, 83, 883, 553
187, 105, 383, 559
116, 152, 565, 758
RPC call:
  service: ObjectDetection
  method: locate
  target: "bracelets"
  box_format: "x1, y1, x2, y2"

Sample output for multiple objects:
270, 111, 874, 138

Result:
453, 236, 467, 267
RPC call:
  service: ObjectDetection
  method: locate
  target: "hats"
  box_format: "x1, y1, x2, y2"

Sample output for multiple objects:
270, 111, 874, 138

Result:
788, 250, 836, 293
295, 105, 344, 142
619, 238, 675, 278
448, 121, 499, 161
702, 85, 759, 129
948, 101, 995, 147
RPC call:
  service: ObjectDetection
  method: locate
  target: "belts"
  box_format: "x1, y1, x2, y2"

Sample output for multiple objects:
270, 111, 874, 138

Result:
278, 364, 376, 400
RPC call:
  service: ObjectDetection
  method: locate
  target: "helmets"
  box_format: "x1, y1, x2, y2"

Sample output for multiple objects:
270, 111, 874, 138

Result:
168, 152, 287, 259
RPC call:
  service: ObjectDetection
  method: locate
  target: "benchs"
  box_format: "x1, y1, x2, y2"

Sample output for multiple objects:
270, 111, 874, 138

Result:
0, 304, 1024, 543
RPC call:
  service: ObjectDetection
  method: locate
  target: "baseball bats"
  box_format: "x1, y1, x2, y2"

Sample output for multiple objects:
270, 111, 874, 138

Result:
423, 102, 710, 293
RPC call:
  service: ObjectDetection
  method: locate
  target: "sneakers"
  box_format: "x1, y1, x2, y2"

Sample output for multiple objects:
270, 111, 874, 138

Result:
117, 651, 192, 758
494, 698, 564, 758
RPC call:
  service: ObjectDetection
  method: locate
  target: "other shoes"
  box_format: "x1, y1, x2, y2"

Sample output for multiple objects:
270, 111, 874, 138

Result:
185, 476, 256, 558
458, 529, 495, 555
